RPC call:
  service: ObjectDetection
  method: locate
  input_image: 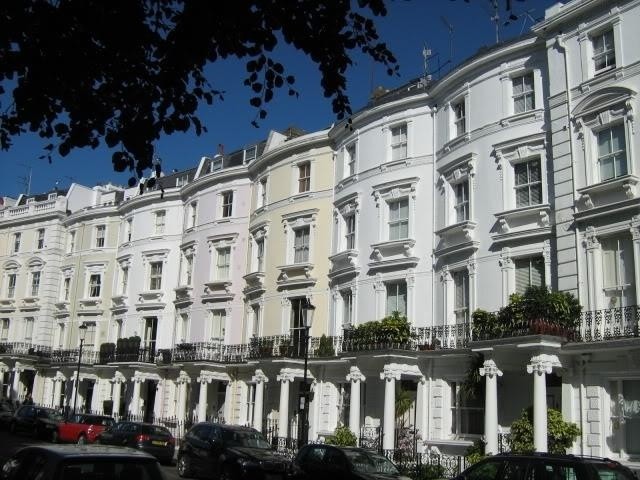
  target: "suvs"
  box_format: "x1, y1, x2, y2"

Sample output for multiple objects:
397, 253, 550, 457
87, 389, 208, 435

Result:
177, 422, 292, 480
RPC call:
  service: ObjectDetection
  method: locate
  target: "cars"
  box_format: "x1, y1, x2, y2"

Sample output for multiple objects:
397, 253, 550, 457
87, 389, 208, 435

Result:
94, 421, 175, 465
52, 414, 116, 444
0, 443, 168, 479
451, 451, 637, 480
9, 405, 64, 438
290, 443, 411, 480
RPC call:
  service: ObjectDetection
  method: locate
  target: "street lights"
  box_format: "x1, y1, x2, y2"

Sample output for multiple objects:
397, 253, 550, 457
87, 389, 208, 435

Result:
301, 297, 316, 446
72, 324, 87, 414
